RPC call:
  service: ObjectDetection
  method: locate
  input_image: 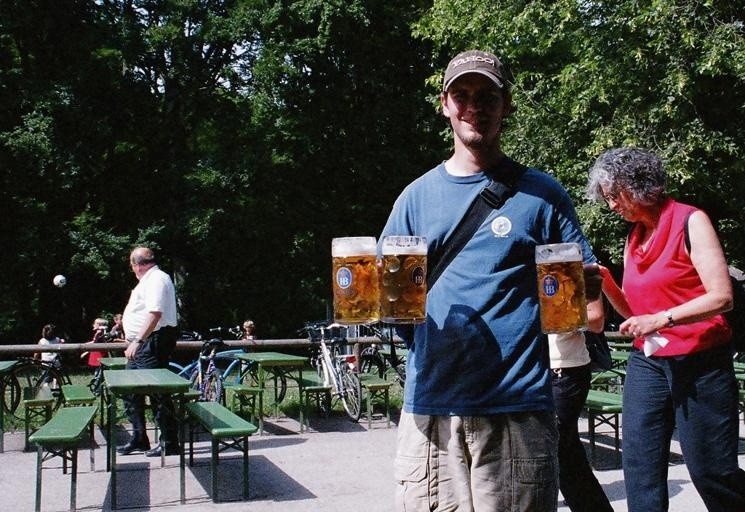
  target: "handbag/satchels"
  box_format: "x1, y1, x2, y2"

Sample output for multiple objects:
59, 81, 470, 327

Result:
719, 270, 745, 356
583, 328, 615, 374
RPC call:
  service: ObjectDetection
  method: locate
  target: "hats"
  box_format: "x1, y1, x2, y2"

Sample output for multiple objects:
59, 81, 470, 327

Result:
442, 49, 509, 94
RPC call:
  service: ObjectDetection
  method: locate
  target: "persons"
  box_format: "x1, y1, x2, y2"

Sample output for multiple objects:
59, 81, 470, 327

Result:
548, 284, 616, 512
108, 313, 125, 359
80, 317, 108, 396
31, 324, 64, 391
582, 144, 745, 511
116, 246, 180, 457
241, 320, 258, 382
369, 50, 604, 512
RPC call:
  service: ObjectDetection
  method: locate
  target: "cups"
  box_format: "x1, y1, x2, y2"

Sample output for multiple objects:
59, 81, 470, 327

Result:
381, 233, 428, 326
329, 236, 381, 327
535, 243, 590, 336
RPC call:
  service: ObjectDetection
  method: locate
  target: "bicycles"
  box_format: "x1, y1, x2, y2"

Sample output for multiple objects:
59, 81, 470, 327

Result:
295, 322, 362, 421
168, 324, 287, 408
360, 322, 408, 388
1, 332, 124, 424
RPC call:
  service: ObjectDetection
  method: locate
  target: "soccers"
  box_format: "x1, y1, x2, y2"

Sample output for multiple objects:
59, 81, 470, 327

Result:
54, 275, 66, 287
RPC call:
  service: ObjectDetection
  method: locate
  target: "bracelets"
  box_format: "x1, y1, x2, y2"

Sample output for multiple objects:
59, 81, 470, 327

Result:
664, 310, 675, 327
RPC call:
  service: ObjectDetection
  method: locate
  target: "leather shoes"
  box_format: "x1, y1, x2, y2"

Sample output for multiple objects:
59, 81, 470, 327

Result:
115, 439, 152, 455
146, 438, 182, 457
